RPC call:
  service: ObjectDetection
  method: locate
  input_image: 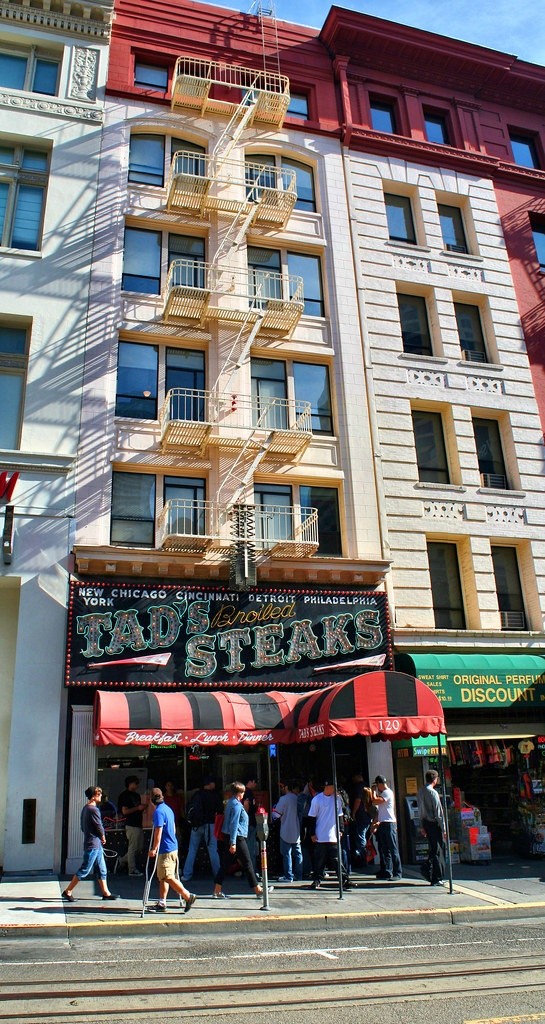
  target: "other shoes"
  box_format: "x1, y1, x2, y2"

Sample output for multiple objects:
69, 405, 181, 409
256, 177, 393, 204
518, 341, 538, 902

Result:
184, 893, 197, 912
212, 891, 230, 900
256, 886, 275, 898
129, 870, 144, 877
342, 881, 358, 890
389, 875, 402, 881
62, 891, 77, 902
278, 876, 293, 882
180, 875, 188, 881
309, 881, 320, 889
431, 881, 444, 886
102, 893, 121, 900
146, 900, 166, 912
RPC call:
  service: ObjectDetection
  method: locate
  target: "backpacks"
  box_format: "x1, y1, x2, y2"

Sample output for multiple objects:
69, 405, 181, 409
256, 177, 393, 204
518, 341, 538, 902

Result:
184, 790, 204, 826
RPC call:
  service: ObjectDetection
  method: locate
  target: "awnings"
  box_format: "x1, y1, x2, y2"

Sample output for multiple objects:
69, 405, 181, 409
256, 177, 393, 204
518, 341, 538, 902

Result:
91, 689, 299, 872
292, 670, 453, 894
407, 652, 545, 710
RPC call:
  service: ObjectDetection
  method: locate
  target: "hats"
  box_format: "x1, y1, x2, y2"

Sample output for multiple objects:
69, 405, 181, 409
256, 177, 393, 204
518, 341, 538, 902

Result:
320, 777, 333, 787
372, 775, 386, 784
151, 787, 162, 799
350, 768, 363, 776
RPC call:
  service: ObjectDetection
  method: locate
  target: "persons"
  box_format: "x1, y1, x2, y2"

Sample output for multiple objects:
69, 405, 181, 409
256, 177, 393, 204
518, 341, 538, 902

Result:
416, 770, 449, 888
241, 776, 263, 882
180, 776, 224, 884
271, 779, 360, 892
62, 786, 119, 901
140, 790, 158, 850
99, 794, 117, 822
145, 788, 197, 912
115, 775, 146, 877
161, 782, 185, 834
350, 774, 402, 881
212, 779, 264, 899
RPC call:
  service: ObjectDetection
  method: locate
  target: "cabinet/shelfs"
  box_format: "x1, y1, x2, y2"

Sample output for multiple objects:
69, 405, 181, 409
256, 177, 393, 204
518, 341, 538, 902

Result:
470, 775, 513, 826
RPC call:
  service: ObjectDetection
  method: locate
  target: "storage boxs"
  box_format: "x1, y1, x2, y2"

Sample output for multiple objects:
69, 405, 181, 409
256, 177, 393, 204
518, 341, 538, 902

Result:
450, 806, 492, 863
